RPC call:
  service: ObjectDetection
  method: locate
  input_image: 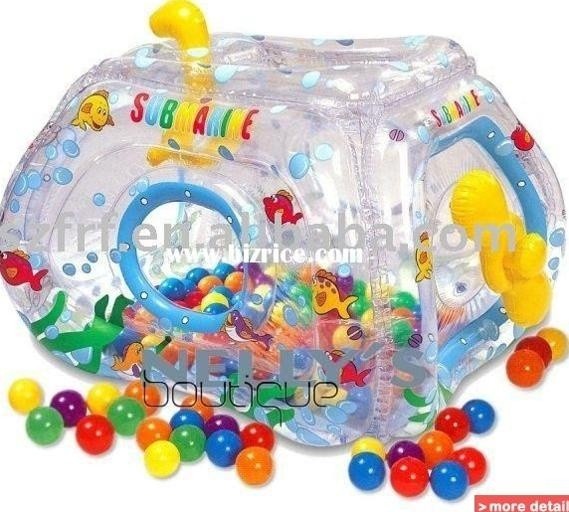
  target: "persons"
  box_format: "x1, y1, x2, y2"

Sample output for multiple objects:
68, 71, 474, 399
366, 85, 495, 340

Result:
10, 262, 568, 500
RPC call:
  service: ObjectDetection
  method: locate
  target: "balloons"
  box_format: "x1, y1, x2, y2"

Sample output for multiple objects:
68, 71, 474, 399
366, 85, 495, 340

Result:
10, 262, 568, 500
